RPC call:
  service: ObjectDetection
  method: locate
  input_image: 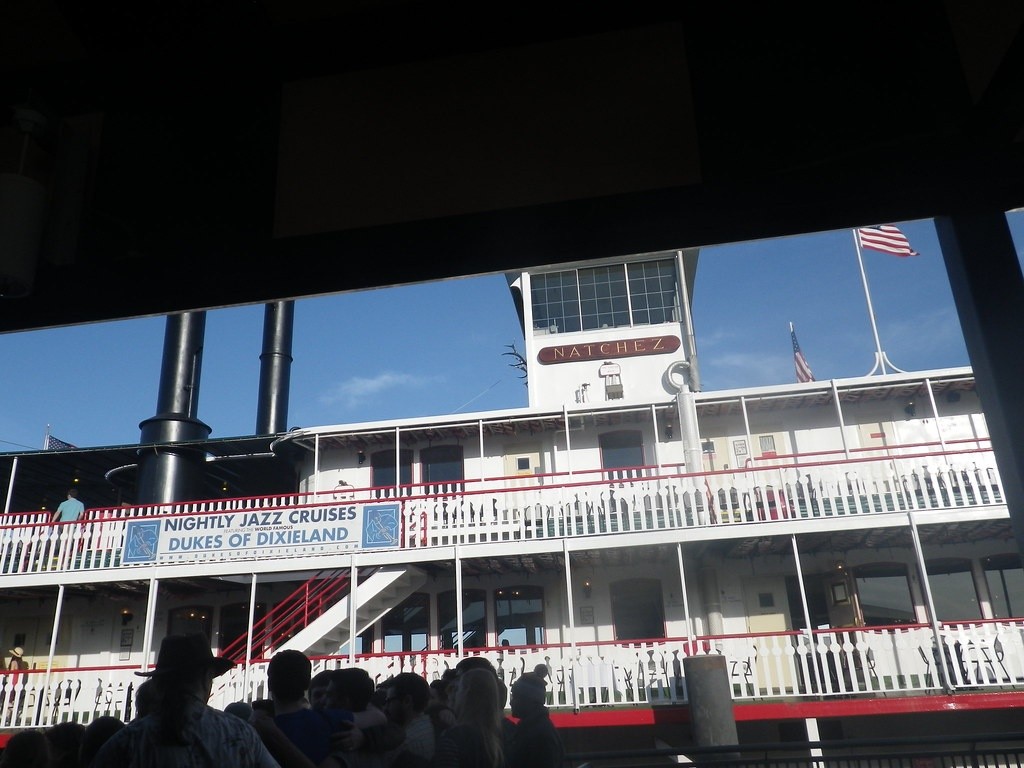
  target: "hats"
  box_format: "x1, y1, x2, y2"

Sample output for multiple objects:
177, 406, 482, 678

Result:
134, 631, 235, 680
512, 664, 548, 708
9, 646, 23, 658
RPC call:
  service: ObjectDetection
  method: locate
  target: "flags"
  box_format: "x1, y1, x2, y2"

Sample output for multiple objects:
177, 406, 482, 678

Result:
858, 225, 920, 257
791, 331, 815, 383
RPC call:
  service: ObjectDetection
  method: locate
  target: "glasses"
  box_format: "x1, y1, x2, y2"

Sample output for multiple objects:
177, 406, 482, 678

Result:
510, 693, 521, 701
383, 696, 404, 705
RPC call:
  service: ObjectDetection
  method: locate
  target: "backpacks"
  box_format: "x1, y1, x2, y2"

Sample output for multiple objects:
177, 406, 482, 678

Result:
16, 661, 28, 670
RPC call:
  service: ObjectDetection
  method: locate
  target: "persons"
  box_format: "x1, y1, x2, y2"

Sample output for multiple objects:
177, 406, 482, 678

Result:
0, 630, 563, 768
52, 489, 84, 568
0, 647, 23, 721
501, 639, 509, 647
